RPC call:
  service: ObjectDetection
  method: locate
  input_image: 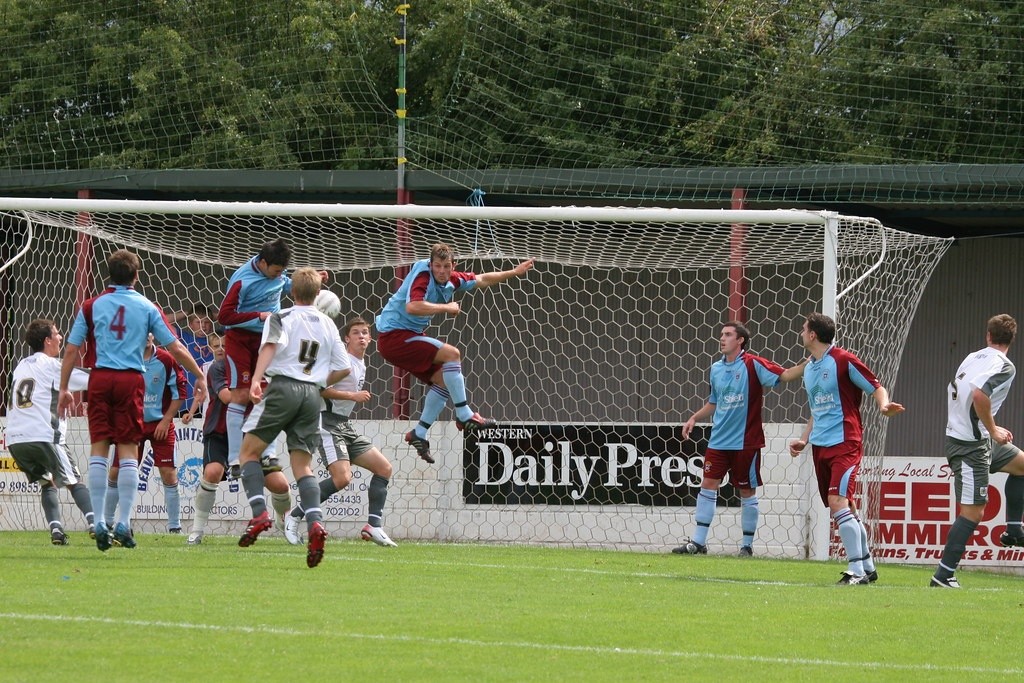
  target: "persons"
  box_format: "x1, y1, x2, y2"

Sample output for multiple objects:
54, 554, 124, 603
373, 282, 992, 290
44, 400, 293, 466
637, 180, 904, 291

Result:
57, 250, 207, 550
166, 304, 214, 418
930, 314, 1024, 587
238, 268, 353, 568
789, 315, 906, 585
375, 245, 536, 463
672, 323, 813, 557
285, 318, 398, 547
5, 319, 122, 547
182, 240, 329, 544
105, 333, 188, 534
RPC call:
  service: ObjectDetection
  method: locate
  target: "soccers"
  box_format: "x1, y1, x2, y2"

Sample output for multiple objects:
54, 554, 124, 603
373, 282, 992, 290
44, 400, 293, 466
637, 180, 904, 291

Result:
313, 289, 341, 321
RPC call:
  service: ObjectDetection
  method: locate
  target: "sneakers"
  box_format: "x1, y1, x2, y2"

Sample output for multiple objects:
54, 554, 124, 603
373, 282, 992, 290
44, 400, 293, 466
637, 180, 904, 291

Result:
275, 518, 285, 533
186, 532, 202, 544
113, 522, 136, 548
89, 524, 96, 539
307, 522, 328, 568
931, 577, 960, 588
672, 541, 707, 555
52, 528, 69, 545
239, 510, 272, 547
96, 522, 112, 551
169, 528, 181, 534
405, 429, 435, 463
1000, 532, 1024, 547
284, 513, 301, 546
838, 573, 869, 584
864, 570, 877, 581
456, 412, 496, 431
361, 524, 398, 547
739, 547, 753, 557
